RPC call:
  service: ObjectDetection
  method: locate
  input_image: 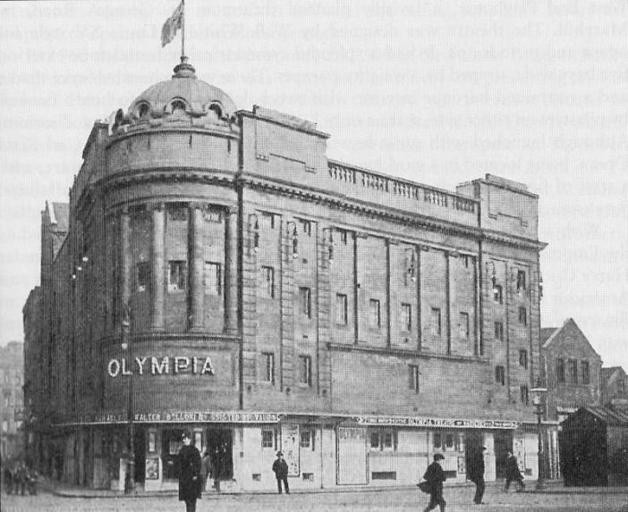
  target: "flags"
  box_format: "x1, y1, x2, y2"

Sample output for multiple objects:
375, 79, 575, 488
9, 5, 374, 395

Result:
159, 1, 187, 47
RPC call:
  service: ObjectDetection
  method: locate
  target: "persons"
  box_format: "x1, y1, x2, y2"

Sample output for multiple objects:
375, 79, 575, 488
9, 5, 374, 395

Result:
174, 431, 203, 512
2, 457, 41, 498
467, 445, 489, 503
502, 450, 527, 493
421, 453, 447, 512
201, 451, 210, 490
272, 450, 290, 495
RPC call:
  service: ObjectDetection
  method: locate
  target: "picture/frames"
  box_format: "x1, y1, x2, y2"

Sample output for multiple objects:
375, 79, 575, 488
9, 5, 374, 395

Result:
512, 437, 526, 472
280, 422, 300, 477
455, 435, 464, 453
145, 457, 159, 480
194, 430, 203, 453
147, 432, 157, 454
457, 455, 467, 474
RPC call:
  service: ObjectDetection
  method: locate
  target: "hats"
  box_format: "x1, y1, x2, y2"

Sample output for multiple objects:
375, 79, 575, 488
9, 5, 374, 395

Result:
434, 453, 445, 460
276, 451, 283, 456
